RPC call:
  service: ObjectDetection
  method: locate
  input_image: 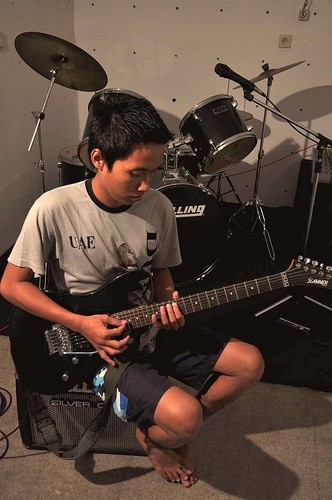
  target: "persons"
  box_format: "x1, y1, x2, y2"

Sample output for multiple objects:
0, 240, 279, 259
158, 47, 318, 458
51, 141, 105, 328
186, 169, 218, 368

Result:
0, 93, 265, 487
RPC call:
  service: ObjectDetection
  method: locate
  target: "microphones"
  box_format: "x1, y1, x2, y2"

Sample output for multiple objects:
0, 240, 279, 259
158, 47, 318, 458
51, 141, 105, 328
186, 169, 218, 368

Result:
215, 64, 266, 98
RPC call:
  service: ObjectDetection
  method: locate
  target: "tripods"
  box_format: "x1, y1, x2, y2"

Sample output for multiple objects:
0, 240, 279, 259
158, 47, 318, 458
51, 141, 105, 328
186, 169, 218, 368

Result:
228, 76, 275, 263
243, 91, 332, 319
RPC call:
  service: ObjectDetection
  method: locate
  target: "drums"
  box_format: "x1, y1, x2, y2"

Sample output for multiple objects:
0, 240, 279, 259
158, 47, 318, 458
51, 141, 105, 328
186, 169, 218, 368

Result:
57, 145, 96, 186
77, 88, 152, 173
180, 94, 257, 173
148, 173, 225, 288
165, 145, 201, 180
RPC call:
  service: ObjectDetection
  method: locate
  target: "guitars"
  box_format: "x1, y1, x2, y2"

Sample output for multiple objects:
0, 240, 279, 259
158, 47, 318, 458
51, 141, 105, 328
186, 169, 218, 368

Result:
9, 256, 332, 394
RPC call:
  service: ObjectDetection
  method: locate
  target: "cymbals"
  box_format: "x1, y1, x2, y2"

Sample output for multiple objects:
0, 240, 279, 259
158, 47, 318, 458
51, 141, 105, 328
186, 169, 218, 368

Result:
14, 32, 108, 92
237, 110, 254, 121
233, 60, 305, 90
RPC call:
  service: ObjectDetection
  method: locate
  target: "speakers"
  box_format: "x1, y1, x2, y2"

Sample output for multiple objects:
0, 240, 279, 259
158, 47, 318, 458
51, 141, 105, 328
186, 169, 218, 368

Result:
289, 159, 332, 267
16, 376, 148, 457
